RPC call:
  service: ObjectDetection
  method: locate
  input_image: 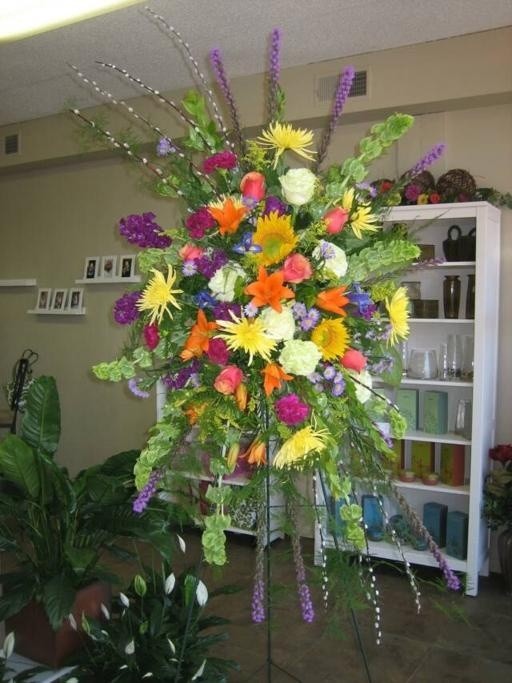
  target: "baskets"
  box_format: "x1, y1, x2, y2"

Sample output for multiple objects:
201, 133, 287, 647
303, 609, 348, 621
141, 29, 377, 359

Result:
442, 223, 476, 263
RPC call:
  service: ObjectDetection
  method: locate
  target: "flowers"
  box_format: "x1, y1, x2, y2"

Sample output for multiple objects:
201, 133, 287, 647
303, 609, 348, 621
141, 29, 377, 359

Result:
67, 5, 464, 648
481, 442, 512, 536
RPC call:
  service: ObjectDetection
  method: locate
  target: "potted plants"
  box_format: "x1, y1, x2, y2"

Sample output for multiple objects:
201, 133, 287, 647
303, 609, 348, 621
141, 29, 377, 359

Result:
0, 377, 192, 671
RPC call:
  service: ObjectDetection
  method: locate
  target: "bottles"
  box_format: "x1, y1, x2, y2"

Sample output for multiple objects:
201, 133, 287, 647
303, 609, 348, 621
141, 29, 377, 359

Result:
454, 399, 471, 437
400, 282, 422, 318
465, 273, 475, 320
398, 341, 409, 378
438, 333, 474, 382
442, 274, 461, 319
407, 349, 438, 380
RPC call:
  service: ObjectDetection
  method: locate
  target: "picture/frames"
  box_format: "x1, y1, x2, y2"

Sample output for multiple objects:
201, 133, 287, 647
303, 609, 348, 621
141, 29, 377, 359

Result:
26, 253, 143, 316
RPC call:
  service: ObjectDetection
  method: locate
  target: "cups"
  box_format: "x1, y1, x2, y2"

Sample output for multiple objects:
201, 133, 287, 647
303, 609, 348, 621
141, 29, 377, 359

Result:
412, 299, 439, 318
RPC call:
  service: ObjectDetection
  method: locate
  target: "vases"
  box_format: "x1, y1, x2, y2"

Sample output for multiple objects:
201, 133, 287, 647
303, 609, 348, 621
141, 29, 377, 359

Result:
495, 524, 512, 594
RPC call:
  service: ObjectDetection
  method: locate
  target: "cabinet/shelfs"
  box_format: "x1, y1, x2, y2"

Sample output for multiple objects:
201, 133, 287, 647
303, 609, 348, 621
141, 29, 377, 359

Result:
313, 199, 503, 599
156, 369, 288, 551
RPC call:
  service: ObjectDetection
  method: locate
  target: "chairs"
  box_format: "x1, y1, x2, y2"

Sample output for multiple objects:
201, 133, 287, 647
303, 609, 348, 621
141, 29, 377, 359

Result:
0, 358, 30, 477
